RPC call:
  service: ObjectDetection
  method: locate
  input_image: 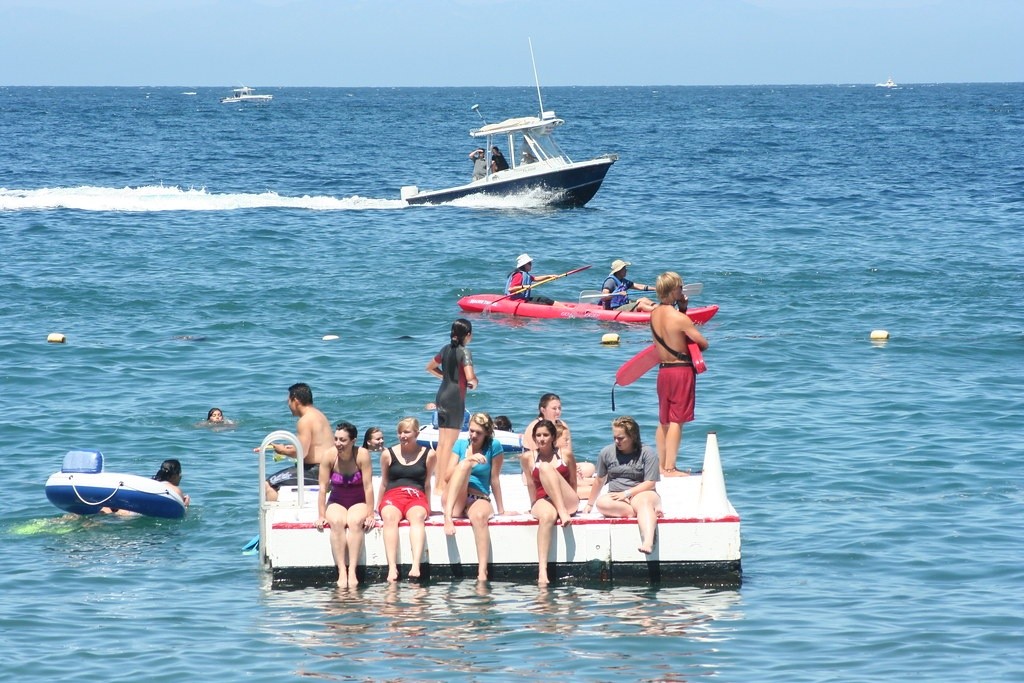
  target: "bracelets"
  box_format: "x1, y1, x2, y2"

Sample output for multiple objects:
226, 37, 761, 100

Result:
624, 490, 631, 498
473, 150, 478, 153
501, 510, 505, 514
680, 307, 687, 313
644, 285, 649, 291
587, 503, 593, 506
521, 285, 524, 289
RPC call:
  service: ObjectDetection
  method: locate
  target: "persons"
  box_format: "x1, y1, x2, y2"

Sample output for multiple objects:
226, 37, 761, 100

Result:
521, 420, 580, 583
197, 408, 234, 433
583, 416, 664, 554
507, 254, 565, 309
599, 259, 658, 311
469, 149, 488, 181
377, 417, 437, 582
520, 394, 601, 499
650, 271, 708, 477
317, 422, 374, 590
492, 414, 514, 432
424, 401, 436, 410
490, 145, 509, 173
261, 383, 334, 503
427, 319, 479, 494
519, 137, 534, 166
362, 427, 389, 451
441, 412, 521, 581
50, 459, 190, 527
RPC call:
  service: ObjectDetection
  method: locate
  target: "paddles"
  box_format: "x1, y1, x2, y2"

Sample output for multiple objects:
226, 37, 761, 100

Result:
579, 283, 704, 304
491, 264, 593, 305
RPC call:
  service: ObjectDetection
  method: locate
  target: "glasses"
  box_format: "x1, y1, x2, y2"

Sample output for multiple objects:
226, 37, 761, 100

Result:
621, 267, 627, 271
528, 262, 531, 266
675, 286, 682, 290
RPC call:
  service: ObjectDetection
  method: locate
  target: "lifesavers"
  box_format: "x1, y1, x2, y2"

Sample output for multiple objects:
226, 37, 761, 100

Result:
615, 334, 707, 387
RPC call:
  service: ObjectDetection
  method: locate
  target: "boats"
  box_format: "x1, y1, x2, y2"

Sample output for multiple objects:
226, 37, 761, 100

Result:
876, 78, 895, 87
400, 36, 619, 207
458, 294, 718, 326
221, 85, 273, 103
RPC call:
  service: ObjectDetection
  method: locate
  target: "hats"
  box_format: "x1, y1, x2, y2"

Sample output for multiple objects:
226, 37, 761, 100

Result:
515, 253, 533, 270
609, 260, 631, 276
